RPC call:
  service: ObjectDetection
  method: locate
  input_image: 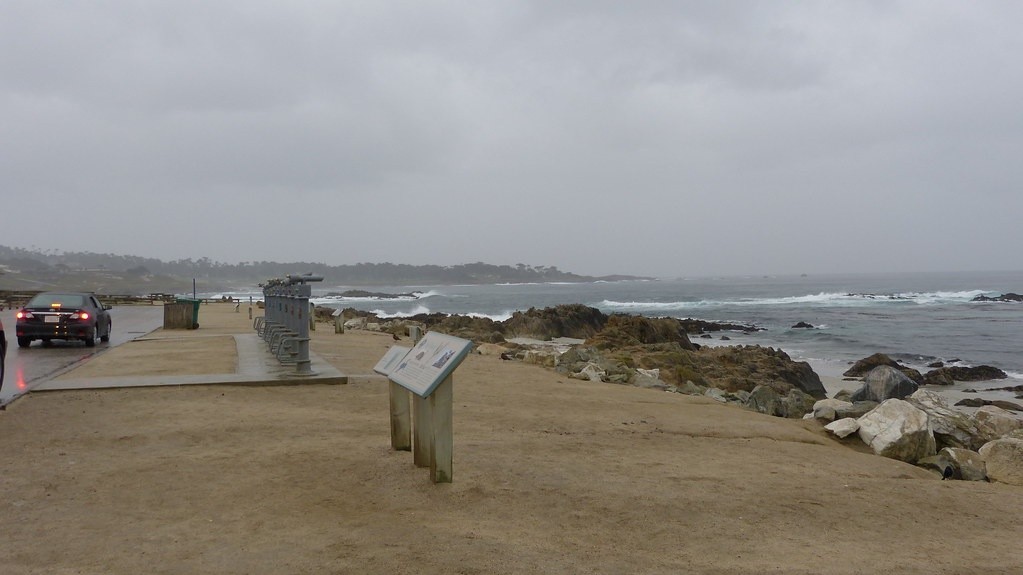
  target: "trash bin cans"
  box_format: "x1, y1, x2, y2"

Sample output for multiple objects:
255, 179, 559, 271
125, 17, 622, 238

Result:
176, 298, 201, 329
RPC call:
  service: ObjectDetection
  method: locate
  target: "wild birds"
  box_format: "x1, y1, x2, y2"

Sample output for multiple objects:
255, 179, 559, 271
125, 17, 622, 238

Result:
393, 334, 402, 341
941, 465, 954, 480
501, 352, 512, 361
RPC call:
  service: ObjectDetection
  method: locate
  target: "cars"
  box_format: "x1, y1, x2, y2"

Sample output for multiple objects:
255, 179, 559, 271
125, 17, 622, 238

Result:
14, 291, 112, 347
